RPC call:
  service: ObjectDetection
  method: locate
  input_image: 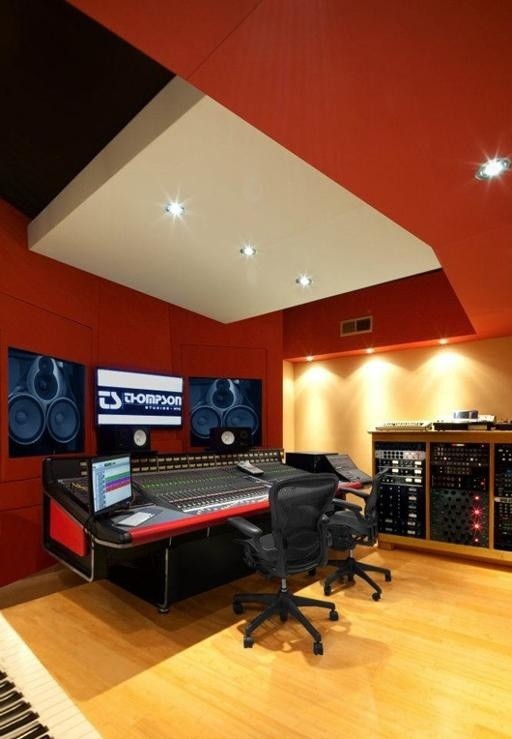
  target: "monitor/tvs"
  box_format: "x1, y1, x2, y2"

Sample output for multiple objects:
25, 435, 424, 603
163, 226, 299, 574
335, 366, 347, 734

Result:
95, 367, 184, 430
88, 453, 133, 516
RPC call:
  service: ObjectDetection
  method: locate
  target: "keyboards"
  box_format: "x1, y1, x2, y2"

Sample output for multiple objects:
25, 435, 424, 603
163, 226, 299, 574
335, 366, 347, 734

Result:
118, 512, 155, 526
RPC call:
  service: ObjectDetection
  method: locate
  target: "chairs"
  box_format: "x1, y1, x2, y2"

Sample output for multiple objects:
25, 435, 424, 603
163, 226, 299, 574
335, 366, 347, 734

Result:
305, 464, 395, 600
230, 470, 345, 657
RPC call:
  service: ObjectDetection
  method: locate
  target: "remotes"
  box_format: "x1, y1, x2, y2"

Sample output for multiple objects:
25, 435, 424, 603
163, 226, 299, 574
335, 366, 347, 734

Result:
237, 462, 264, 475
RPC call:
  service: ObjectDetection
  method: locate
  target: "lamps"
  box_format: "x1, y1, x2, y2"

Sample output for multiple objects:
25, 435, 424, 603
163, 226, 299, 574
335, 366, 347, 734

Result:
295, 275, 311, 288
162, 202, 187, 217
239, 245, 256, 258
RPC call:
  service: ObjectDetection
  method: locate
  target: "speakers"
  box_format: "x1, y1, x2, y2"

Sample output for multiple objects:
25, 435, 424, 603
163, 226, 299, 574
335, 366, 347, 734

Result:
96, 424, 152, 456
189, 377, 262, 448
8, 345, 85, 458
210, 427, 251, 453
